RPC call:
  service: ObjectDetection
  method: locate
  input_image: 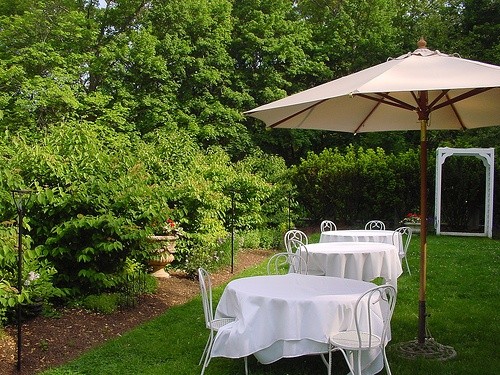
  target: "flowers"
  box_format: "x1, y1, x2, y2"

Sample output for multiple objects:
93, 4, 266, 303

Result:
398, 207, 434, 224
152, 218, 180, 236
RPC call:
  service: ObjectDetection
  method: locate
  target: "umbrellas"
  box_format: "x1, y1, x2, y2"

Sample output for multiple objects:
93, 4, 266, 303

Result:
242, 38, 500, 342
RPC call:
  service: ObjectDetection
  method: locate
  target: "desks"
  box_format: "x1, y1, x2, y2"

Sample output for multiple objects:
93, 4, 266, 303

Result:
210, 274, 391, 375
319, 229, 402, 263
287, 241, 403, 292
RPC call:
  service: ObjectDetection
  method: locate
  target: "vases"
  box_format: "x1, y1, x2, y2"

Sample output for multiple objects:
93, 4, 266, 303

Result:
404, 222, 422, 237
144, 235, 179, 278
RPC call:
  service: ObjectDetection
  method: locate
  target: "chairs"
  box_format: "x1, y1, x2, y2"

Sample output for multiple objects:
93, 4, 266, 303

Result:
329, 285, 397, 375
198, 267, 248, 375
365, 220, 385, 230
394, 227, 412, 276
266, 221, 338, 276
